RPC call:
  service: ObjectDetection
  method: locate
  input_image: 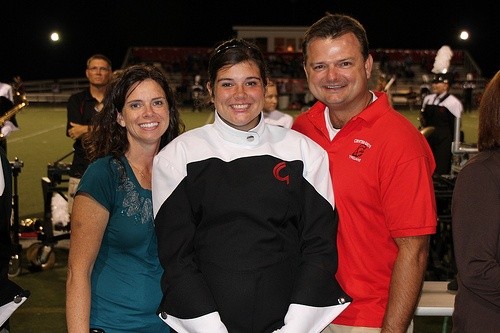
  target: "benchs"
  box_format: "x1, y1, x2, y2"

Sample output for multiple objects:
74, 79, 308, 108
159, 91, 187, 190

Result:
414, 281, 458, 333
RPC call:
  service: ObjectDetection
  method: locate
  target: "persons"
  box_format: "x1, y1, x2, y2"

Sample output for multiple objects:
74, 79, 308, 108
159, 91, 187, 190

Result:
0, 81, 19, 153
151, 39, 353, 333
65, 54, 111, 222
0, 133, 32, 333
300, 82, 318, 112
451, 71, 499, 333
264, 81, 293, 130
290, 14, 437, 333
419, 74, 431, 107
190, 75, 204, 112
65, 65, 188, 333
461, 74, 476, 112
406, 86, 420, 110
419, 46, 463, 176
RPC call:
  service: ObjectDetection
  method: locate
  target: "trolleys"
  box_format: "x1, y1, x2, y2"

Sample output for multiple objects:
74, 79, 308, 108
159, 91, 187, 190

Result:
25, 174, 76, 274
5, 157, 44, 278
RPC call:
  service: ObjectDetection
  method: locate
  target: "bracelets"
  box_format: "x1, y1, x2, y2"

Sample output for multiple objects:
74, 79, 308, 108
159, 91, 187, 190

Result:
88, 125, 91, 133
